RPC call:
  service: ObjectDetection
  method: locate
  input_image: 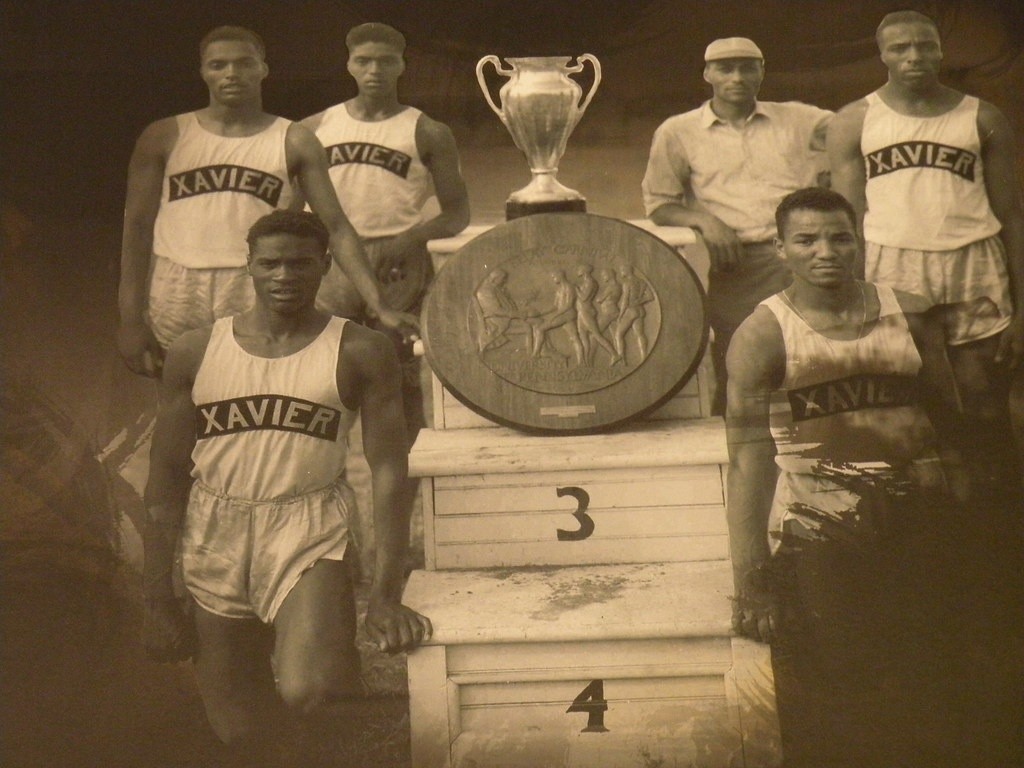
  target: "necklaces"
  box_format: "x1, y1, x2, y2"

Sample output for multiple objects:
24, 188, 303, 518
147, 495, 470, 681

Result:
782, 279, 866, 352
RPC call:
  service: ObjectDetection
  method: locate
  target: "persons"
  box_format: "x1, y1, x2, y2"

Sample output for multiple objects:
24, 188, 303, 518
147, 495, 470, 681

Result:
725, 188, 969, 768
144, 208, 433, 768
642, 37, 837, 417
287, 20, 472, 450
116, 26, 421, 413
828, 11, 1024, 514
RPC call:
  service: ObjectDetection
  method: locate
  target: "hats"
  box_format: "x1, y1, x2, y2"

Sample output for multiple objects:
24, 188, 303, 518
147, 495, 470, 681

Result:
704, 37, 762, 66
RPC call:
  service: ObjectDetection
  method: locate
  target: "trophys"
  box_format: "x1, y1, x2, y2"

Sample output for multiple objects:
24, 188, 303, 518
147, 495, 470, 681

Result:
476, 52, 602, 222
